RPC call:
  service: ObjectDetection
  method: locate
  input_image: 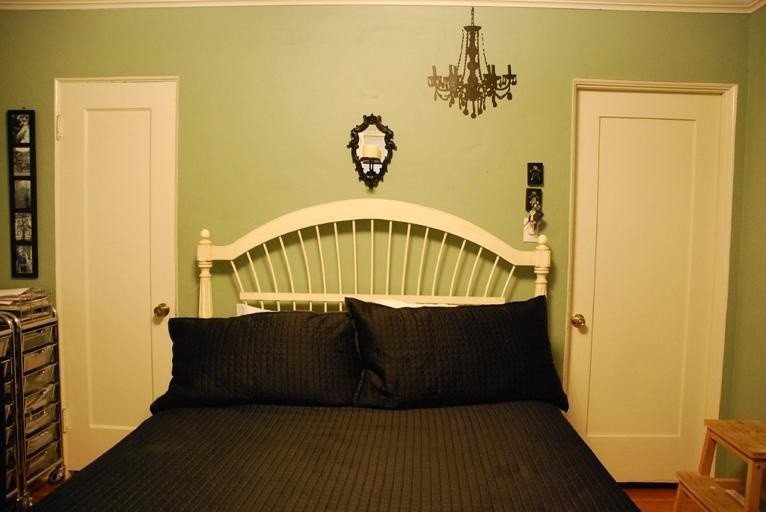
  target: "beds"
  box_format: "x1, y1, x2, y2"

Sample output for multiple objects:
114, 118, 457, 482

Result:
25, 199, 642, 512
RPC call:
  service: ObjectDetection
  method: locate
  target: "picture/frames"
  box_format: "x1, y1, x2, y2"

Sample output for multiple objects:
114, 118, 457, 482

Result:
6, 109, 39, 280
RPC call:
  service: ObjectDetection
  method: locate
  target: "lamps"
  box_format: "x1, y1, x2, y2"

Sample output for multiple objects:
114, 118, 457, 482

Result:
427, 5, 517, 119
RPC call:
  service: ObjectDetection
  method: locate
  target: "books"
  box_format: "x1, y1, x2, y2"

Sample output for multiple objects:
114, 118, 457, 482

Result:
0, 285, 49, 310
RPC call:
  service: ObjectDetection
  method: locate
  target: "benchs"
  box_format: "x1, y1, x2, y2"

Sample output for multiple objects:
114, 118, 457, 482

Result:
674, 469, 744, 512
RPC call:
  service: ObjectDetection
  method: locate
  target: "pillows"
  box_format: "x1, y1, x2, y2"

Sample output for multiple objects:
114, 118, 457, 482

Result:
345, 295, 569, 414
150, 303, 360, 414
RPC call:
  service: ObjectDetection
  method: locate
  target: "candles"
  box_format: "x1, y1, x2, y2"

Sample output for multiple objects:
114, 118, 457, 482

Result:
360, 143, 382, 159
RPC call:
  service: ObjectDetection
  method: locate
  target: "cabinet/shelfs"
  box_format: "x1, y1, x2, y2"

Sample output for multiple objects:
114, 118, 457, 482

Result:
0, 306, 66, 512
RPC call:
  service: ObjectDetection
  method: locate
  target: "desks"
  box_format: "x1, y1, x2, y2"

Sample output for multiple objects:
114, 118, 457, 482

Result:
699, 419, 765, 510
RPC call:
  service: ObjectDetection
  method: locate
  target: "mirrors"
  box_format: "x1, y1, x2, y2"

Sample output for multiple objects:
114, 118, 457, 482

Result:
346, 114, 396, 192
